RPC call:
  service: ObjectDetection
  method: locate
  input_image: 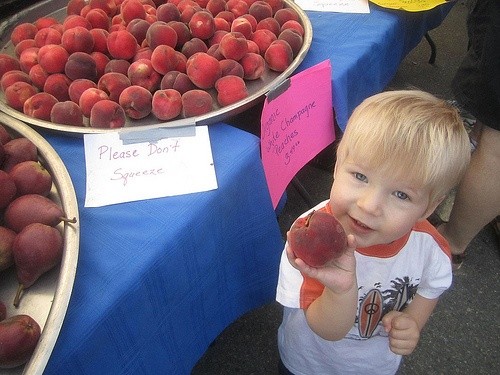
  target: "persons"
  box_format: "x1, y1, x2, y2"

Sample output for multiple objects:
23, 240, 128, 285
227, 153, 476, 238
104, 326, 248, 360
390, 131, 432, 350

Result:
428, 0, 500, 269
276, 89, 471, 375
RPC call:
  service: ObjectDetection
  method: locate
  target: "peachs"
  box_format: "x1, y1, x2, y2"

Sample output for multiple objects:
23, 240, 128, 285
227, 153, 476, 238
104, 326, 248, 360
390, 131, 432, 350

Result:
290, 211, 346, 268
0, 0, 305, 129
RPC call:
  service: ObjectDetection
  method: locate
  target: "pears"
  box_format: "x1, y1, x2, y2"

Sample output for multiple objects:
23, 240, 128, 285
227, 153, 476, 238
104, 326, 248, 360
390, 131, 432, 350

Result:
0, 119, 78, 369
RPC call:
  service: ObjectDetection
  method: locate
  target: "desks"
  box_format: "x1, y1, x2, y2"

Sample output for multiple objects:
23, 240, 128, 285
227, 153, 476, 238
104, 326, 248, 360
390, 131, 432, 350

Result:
239, 0, 461, 175
25, 122, 286, 375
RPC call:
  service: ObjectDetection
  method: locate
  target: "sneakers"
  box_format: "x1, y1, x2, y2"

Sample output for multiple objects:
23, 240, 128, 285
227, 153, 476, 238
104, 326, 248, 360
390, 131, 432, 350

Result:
441, 99, 476, 127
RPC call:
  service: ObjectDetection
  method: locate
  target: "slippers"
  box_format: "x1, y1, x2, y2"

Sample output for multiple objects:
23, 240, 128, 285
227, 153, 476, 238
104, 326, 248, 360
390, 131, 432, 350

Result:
426, 211, 462, 271
